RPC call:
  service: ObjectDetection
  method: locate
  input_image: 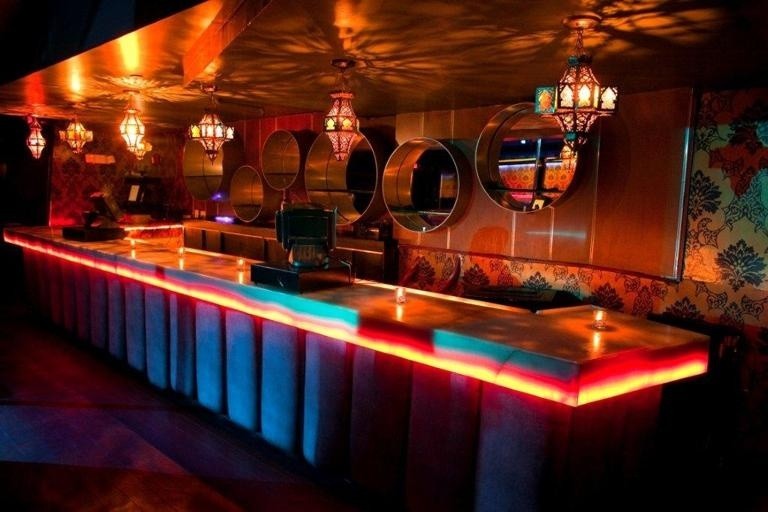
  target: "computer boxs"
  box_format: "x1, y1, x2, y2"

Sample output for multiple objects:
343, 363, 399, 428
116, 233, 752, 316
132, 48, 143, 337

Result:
63, 226, 125, 242
251, 260, 356, 294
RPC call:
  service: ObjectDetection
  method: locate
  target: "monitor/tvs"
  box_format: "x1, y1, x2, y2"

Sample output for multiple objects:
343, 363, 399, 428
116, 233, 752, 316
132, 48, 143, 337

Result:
83, 191, 118, 229
274, 202, 337, 267
